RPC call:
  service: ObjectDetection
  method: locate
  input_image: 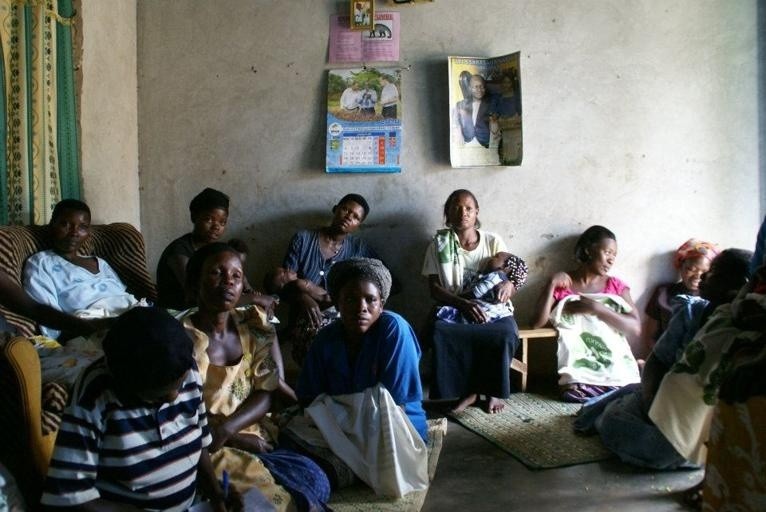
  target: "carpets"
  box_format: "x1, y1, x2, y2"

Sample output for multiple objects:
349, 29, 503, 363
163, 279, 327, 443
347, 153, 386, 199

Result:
325, 416, 447, 512
449, 388, 615, 470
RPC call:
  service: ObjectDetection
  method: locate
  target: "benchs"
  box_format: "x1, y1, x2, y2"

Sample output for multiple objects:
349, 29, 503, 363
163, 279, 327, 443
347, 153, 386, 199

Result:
509, 325, 559, 390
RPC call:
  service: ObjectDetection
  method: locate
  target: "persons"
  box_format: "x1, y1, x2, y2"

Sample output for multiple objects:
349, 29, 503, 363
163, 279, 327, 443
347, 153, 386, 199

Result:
171, 238, 331, 512
261, 192, 371, 367
531, 215, 766, 512
453, 68, 524, 166
339, 80, 363, 113
262, 265, 327, 305
354, 2, 363, 25
420, 188, 520, 415
39, 305, 214, 512
379, 73, 400, 119
362, 8, 371, 25
21, 196, 141, 319
435, 251, 528, 325
154, 185, 231, 308
276, 253, 430, 491
358, 80, 378, 115
228, 237, 265, 296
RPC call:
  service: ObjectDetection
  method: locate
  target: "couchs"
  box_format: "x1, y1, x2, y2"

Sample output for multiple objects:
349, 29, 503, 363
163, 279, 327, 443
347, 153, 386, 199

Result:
0, 325, 70, 496
0, 224, 158, 403
